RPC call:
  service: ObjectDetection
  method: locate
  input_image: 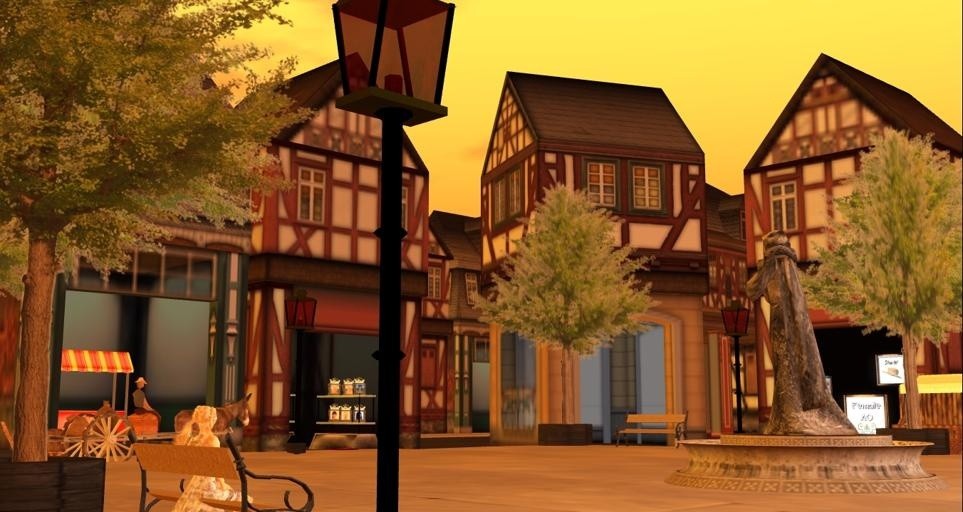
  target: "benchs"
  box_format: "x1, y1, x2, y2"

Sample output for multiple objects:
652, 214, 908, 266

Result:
616, 411, 686, 448
126, 429, 316, 512
